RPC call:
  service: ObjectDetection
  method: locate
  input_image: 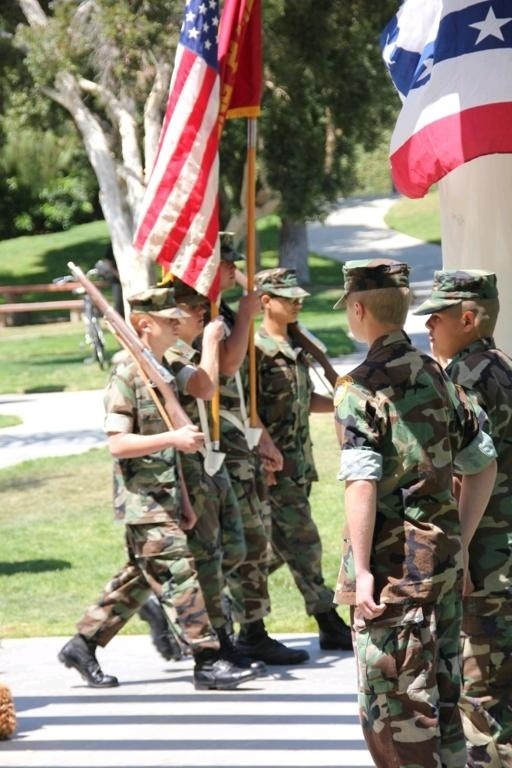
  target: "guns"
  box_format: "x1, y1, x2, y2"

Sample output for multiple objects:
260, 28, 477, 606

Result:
227, 265, 339, 390
67, 259, 195, 435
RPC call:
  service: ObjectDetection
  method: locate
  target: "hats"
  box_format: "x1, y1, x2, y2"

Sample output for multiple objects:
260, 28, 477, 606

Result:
255, 269, 311, 299
333, 259, 409, 311
413, 269, 499, 314
219, 230, 243, 263
127, 288, 190, 320
157, 278, 209, 305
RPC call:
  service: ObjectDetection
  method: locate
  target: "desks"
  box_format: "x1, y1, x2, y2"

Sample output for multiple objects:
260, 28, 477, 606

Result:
0, 281, 110, 324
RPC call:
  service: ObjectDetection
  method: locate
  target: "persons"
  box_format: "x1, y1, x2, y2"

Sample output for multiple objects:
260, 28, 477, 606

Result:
59, 268, 354, 690
412, 270, 512, 768
333, 257, 499, 768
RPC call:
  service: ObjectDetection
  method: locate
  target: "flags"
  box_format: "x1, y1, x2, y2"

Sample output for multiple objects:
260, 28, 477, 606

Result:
217, 0, 262, 144
381, 0, 512, 198
131, 0, 222, 308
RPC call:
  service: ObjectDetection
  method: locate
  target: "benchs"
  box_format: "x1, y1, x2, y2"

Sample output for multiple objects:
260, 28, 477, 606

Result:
1, 299, 86, 327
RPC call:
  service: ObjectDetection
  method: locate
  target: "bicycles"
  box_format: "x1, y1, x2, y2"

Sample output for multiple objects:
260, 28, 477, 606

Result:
56, 267, 110, 370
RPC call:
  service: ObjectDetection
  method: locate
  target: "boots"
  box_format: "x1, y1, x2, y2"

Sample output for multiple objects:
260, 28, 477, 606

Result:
314, 611, 353, 651
194, 621, 308, 690
58, 634, 118, 686
138, 600, 181, 661
